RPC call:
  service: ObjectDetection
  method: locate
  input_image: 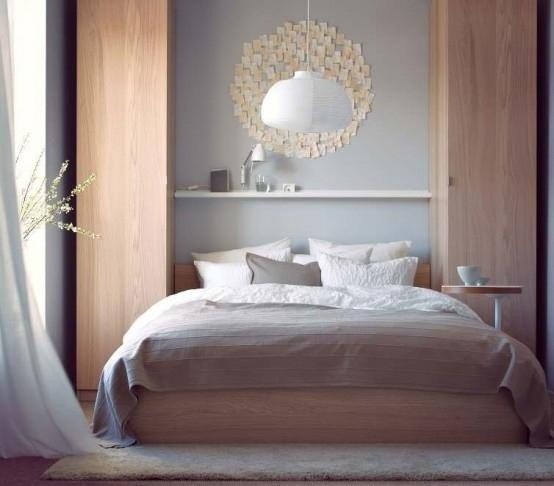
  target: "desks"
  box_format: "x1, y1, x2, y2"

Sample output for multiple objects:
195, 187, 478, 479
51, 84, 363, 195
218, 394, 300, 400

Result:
441, 285, 522, 330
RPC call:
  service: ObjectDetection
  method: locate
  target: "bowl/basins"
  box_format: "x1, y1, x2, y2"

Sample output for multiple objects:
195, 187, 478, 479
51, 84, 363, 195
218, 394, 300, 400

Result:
457, 265, 482, 287
477, 276, 489, 287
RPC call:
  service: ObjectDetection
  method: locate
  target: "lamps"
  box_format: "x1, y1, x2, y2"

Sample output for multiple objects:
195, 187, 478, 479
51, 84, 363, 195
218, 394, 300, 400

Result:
240, 144, 264, 190
261, 0, 352, 134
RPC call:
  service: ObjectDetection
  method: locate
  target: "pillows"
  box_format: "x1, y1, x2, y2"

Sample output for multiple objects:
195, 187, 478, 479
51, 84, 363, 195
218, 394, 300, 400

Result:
293, 252, 312, 265
316, 250, 419, 287
194, 260, 253, 289
246, 251, 321, 286
309, 238, 411, 261
191, 238, 291, 263
328, 247, 372, 264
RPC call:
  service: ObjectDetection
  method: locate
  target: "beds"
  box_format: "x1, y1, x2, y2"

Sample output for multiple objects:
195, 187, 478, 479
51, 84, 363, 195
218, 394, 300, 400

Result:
91, 263, 554, 449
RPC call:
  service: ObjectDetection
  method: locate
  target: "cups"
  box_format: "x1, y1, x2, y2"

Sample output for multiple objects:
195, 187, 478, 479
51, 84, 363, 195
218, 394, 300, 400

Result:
254, 173, 268, 192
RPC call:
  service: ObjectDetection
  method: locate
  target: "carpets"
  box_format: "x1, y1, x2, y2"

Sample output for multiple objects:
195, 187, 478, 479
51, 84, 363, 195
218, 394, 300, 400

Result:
40, 443, 553, 480
